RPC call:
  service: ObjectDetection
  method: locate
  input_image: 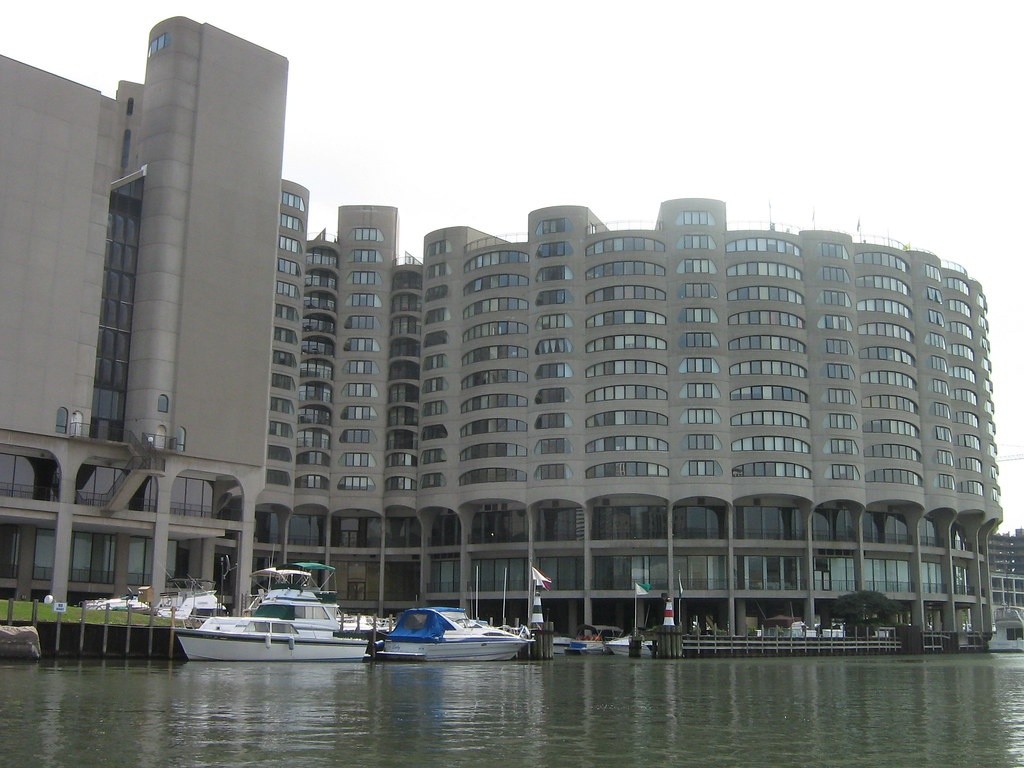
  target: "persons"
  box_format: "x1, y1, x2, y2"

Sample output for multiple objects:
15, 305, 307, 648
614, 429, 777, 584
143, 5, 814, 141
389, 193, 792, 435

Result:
801, 622, 807, 637
815, 625, 823, 637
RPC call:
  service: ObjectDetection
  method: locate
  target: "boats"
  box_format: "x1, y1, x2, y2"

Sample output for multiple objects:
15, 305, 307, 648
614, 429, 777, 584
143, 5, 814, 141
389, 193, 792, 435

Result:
754, 598, 891, 638
336, 565, 658, 662
170, 544, 371, 663
988, 603, 1024, 653
85, 559, 229, 619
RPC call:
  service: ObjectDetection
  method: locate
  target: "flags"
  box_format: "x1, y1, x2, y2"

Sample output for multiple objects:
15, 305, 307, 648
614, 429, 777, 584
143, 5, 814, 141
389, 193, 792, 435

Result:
678, 578, 684, 599
537, 579, 552, 592
636, 583, 652, 596
532, 568, 552, 583
